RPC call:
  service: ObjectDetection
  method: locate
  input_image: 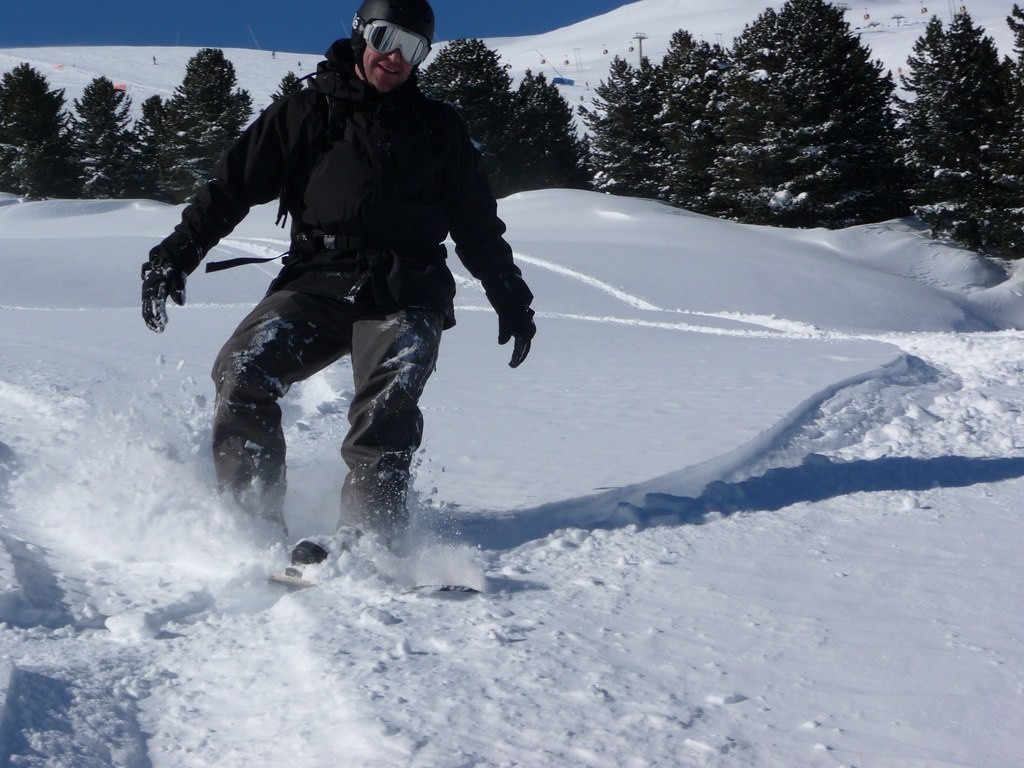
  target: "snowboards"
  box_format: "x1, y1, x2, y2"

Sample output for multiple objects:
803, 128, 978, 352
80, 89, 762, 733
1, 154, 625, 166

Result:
268, 574, 481, 595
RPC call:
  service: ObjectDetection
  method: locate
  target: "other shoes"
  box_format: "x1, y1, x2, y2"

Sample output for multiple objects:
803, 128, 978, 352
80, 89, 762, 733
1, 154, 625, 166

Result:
291, 525, 416, 587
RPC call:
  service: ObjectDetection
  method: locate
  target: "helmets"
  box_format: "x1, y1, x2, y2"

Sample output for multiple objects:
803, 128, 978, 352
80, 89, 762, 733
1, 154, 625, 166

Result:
350, 0, 434, 73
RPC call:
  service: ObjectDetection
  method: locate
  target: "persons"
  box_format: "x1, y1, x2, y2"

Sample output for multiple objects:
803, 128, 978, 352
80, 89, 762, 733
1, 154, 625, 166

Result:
141, 0, 536, 566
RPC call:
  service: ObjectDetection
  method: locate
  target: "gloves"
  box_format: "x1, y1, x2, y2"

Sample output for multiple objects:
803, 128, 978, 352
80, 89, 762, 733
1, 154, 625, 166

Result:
142, 232, 199, 332
481, 256, 536, 369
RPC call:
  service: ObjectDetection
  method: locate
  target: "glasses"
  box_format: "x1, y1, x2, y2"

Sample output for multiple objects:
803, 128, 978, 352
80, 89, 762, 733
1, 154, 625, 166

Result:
366, 20, 428, 65
